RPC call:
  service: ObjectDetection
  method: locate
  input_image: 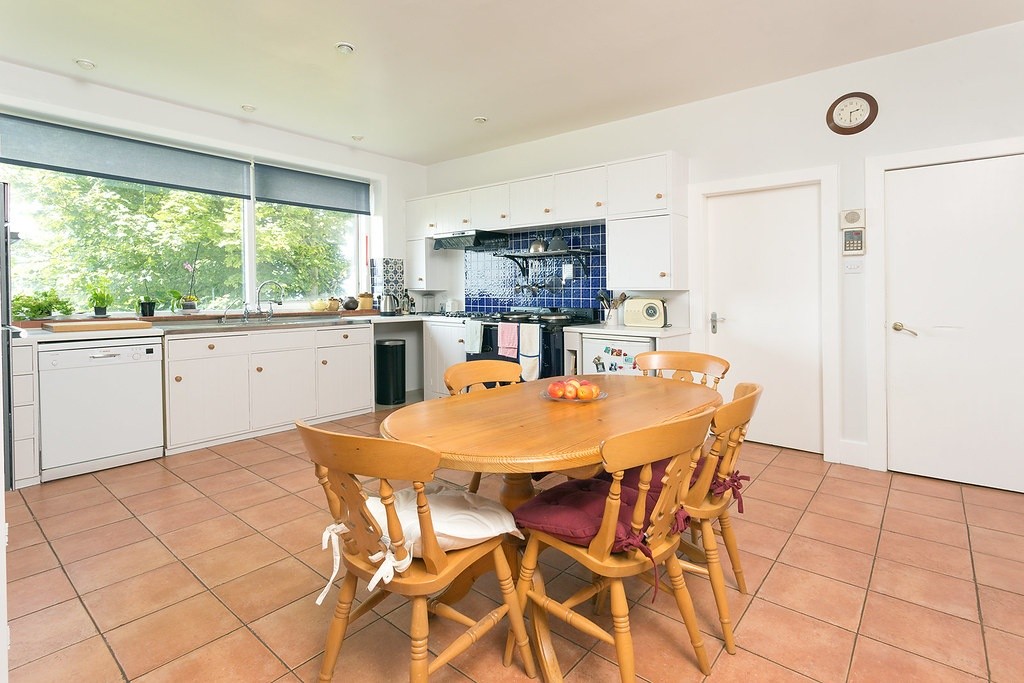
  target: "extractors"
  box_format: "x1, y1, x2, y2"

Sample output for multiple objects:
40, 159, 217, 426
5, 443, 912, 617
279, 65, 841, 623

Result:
433, 230, 508, 251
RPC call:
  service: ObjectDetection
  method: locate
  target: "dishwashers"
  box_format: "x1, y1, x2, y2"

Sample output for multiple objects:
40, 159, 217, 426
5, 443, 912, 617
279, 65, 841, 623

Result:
39, 343, 164, 483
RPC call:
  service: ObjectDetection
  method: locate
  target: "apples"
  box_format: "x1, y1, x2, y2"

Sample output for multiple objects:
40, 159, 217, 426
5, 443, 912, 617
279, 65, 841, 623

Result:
547, 377, 600, 399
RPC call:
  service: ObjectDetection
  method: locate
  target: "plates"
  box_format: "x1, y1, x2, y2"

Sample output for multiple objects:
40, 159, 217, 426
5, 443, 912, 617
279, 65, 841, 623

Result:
540, 390, 608, 402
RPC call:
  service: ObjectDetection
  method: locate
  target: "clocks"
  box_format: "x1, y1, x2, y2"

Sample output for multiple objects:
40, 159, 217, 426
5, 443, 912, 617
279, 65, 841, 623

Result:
825, 92, 879, 135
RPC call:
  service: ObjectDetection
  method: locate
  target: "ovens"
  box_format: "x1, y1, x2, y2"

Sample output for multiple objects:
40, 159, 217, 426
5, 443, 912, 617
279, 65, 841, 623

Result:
466, 322, 564, 393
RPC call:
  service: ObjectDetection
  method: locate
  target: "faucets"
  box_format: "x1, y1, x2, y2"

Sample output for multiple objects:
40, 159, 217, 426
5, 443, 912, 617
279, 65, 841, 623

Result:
256, 280, 284, 313
223, 298, 246, 323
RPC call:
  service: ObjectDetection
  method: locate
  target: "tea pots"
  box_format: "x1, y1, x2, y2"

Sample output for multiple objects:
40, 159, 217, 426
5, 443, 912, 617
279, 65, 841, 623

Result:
308, 296, 358, 311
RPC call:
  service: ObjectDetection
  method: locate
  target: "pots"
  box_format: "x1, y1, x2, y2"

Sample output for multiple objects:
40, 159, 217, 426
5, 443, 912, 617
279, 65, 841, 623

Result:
358, 292, 372, 310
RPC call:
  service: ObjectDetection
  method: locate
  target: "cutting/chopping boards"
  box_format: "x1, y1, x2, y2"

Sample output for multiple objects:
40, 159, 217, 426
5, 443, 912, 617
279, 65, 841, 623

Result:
42, 319, 153, 332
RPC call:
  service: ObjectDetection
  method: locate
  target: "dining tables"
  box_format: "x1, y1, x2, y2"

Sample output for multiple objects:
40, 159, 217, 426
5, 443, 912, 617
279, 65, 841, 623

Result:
380, 374, 722, 617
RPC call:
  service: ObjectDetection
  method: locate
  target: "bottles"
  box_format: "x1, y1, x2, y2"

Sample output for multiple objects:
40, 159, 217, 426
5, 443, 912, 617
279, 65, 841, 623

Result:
422, 294, 435, 315
403, 289, 415, 314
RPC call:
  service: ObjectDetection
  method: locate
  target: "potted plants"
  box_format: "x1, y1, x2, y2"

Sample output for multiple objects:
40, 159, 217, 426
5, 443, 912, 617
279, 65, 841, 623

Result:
166, 288, 201, 314
133, 279, 160, 318
87, 290, 112, 318
11, 287, 76, 323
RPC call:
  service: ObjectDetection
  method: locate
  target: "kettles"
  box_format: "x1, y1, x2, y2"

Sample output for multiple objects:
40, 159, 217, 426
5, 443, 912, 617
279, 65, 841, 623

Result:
530, 227, 569, 252
377, 293, 399, 316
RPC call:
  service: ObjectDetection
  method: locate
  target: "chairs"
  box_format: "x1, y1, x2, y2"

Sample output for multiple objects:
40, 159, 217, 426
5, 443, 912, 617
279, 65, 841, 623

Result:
443, 360, 523, 494
585, 382, 764, 656
632, 352, 731, 391
501, 406, 715, 683
292, 419, 537, 683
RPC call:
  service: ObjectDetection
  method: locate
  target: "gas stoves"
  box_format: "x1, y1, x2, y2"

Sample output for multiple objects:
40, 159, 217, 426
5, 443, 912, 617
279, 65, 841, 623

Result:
445, 311, 491, 318
489, 307, 599, 322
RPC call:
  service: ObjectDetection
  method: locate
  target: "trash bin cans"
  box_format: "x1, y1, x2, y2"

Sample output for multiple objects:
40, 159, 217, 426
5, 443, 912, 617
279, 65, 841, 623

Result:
373, 338, 410, 406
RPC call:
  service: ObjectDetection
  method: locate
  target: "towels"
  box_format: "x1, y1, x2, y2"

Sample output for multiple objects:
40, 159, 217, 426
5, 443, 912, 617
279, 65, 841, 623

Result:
463, 320, 484, 355
497, 321, 519, 359
518, 323, 542, 381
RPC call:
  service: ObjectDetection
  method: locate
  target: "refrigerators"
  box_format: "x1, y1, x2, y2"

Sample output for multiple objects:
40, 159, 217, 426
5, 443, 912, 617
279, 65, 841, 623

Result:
582, 333, 655, 376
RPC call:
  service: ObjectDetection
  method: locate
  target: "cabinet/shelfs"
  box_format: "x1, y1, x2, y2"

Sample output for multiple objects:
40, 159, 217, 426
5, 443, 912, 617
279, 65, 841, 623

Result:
403, 148, 689, 290
422, 315, 472, 402
164, 313, 374, 456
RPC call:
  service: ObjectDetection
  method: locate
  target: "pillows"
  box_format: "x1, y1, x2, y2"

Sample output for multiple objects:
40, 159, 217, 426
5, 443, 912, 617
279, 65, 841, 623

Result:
315, 482, 524, 605
